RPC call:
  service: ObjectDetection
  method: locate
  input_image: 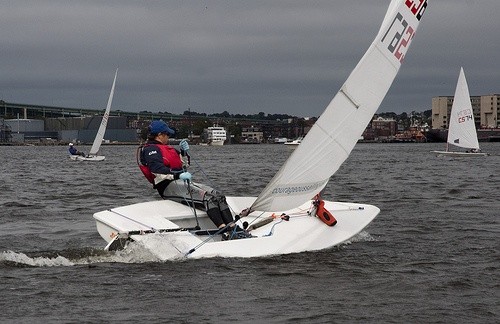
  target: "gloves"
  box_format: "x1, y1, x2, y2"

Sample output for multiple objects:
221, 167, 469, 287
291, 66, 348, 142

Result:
180, 172, 191, 180
179, 139, 189, 152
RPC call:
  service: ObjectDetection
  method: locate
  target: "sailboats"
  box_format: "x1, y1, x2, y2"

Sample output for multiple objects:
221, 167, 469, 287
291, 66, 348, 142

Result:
68, 67, 120, 162
430, 65, 491, 157
93, 0, 429, 262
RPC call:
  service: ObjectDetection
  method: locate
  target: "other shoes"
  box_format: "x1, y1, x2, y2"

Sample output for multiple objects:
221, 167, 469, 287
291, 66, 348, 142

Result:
221, 230, 252, 241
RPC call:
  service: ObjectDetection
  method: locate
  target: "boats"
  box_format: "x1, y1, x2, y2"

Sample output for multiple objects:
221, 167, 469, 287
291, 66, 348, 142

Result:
284, 140, 302, 146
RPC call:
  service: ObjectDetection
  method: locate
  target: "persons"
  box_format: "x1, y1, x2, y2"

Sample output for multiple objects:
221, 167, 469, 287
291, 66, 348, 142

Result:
69, 143, 86, 157
137, 120, 252, 241
470, 148, 478, 153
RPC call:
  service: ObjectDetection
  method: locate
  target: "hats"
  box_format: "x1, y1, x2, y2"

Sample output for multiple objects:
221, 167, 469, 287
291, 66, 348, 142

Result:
149, 121, 175, 135
69, 143, 73, 146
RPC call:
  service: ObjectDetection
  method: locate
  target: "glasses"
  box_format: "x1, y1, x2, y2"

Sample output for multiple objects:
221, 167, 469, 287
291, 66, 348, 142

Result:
160, 132, 169, 136
